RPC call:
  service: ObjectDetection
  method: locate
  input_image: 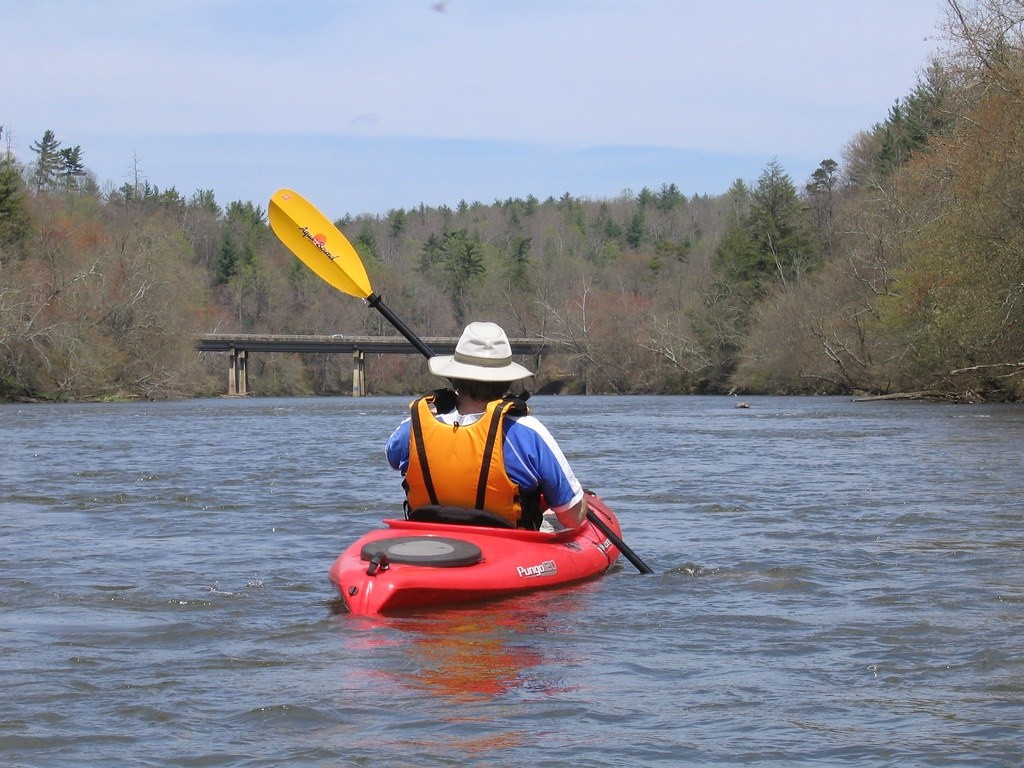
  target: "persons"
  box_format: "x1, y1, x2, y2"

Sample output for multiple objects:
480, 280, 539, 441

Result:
385, 321, 587, 531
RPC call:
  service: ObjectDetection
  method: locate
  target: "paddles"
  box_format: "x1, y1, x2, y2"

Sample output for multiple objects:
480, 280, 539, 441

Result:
272, 191, 650, 573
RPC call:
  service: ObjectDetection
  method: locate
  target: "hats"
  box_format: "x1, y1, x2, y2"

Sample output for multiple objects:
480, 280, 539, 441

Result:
428, 321, 536, 382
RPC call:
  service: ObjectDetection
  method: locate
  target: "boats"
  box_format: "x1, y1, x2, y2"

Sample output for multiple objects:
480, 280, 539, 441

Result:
325, 485, 623, 618
